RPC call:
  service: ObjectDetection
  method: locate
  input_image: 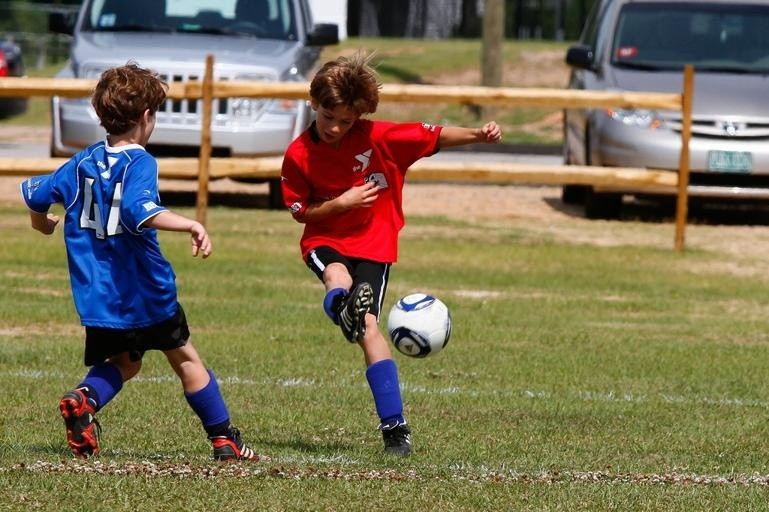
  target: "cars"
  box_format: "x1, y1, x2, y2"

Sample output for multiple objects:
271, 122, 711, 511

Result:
562, 1, 768, 220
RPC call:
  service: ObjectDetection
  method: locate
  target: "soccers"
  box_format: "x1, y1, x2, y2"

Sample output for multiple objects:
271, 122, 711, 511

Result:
387, 292, 452, 358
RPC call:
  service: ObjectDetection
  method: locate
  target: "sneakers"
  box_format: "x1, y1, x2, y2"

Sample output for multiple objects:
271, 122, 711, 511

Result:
209, 426, 258, 460
334, 281, 372, 344
61, 389, 102, 458
378, 419, 411, 456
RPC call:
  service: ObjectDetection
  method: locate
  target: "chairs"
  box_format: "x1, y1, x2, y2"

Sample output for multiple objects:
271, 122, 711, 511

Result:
115, 0, 276, 36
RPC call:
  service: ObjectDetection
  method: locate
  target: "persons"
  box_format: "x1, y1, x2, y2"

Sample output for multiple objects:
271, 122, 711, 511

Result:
279, 48, 504, 460
20, 61, 266, 463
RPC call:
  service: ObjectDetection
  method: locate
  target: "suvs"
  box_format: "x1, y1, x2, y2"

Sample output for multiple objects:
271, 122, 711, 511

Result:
48, 0, 341, 210
